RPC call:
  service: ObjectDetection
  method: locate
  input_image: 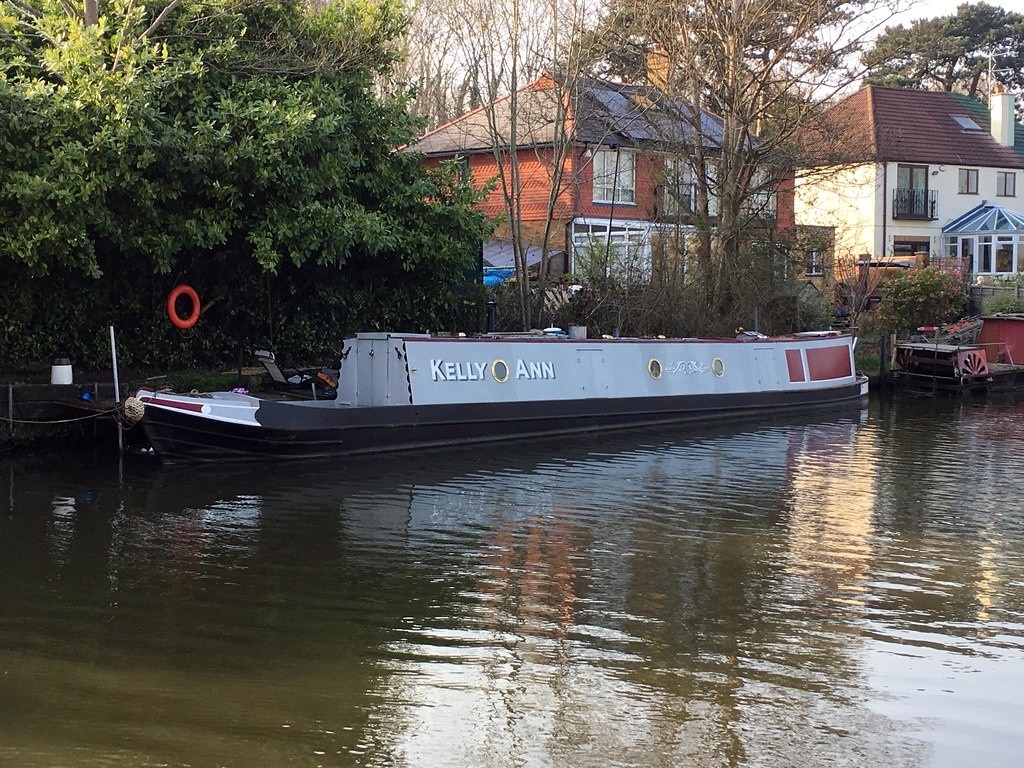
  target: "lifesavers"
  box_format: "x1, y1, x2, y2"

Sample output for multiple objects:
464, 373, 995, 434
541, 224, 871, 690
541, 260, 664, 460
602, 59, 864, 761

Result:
168, 283, 201, 329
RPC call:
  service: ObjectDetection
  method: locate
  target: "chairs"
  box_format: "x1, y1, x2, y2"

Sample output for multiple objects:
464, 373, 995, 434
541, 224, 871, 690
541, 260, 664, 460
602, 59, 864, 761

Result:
257, 358, 315, 388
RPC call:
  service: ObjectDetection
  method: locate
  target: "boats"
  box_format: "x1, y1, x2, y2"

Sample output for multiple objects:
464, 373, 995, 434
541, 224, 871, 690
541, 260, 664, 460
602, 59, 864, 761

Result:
11, 378, 336, 431
124, 328, 872, 456
